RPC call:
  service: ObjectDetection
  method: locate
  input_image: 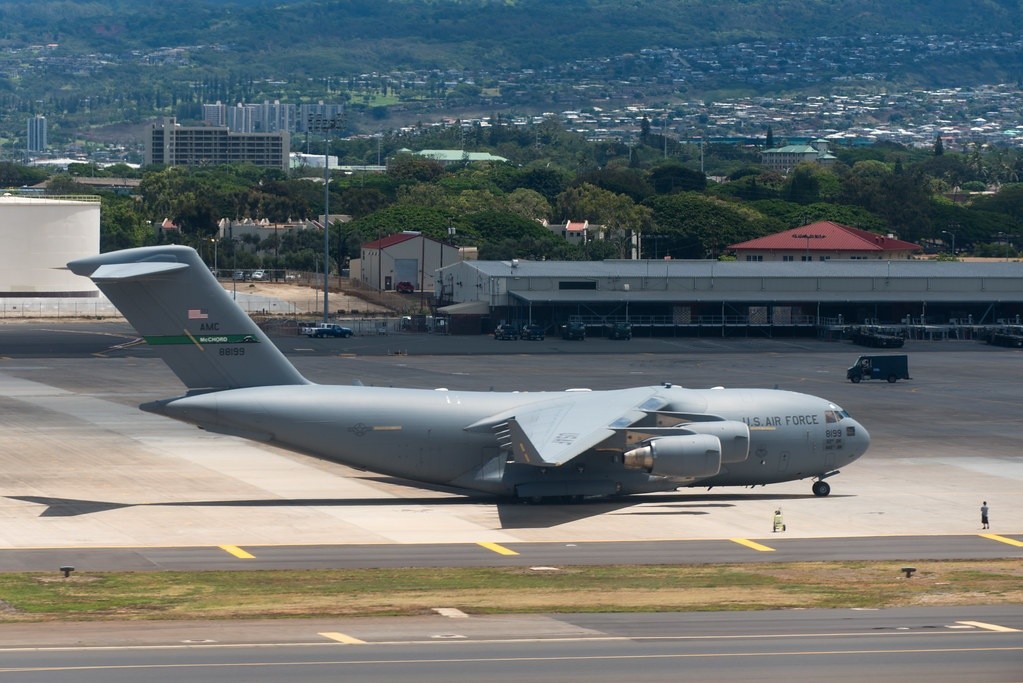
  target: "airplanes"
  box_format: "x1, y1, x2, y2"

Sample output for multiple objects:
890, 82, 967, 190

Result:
67, 243, 872, 503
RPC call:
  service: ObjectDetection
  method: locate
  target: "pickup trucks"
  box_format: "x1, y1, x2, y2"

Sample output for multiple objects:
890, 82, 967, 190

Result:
302, 323, 352, 337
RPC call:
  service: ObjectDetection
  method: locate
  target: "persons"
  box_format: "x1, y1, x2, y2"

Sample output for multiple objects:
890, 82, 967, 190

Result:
981, 501, 989, 528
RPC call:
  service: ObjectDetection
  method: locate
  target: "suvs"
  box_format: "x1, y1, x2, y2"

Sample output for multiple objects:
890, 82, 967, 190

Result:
561, 321, 586, 340
609, 323, 632, 339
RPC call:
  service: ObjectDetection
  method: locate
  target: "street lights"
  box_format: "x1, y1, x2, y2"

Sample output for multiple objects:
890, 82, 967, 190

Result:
306, 109, 347, 325
999, 231, 1009, 262
211, 238, 220, 281
942, 230, 955, 257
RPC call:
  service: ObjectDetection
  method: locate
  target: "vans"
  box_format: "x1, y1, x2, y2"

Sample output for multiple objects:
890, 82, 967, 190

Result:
845, 355, 910, 383
257, 269, 270, 276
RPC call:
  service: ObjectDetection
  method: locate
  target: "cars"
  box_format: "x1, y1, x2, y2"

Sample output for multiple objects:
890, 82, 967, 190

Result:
232, 271, 244, 280
396, 281, 413, 293
493, 323, 518, 340
251, 272, 269, 280
519, 324, 545, 340
